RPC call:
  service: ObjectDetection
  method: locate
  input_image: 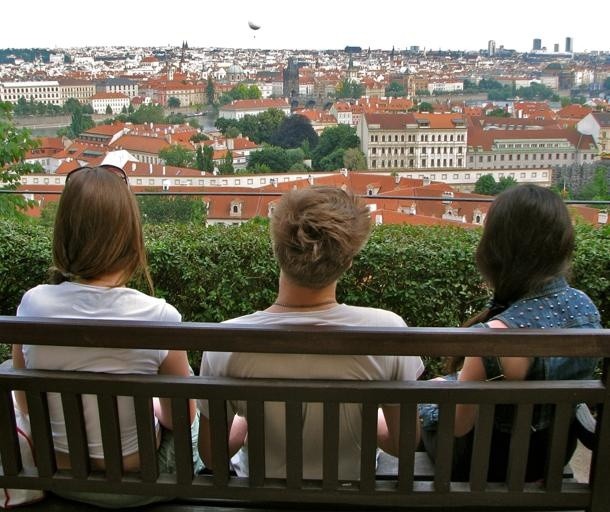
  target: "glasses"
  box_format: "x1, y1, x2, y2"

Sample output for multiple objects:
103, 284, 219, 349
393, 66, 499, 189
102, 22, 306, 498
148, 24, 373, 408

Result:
65, 165, 129, 188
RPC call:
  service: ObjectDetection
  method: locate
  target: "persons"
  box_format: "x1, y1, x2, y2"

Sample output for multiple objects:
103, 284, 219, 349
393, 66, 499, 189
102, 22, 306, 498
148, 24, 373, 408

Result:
1, 164, 198, 474
417, 184, 605, 484
194, 184, 427, 481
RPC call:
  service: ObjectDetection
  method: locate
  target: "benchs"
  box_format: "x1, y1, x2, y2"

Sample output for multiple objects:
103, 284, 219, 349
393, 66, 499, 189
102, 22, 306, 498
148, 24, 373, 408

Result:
0, 315, 610, 512
